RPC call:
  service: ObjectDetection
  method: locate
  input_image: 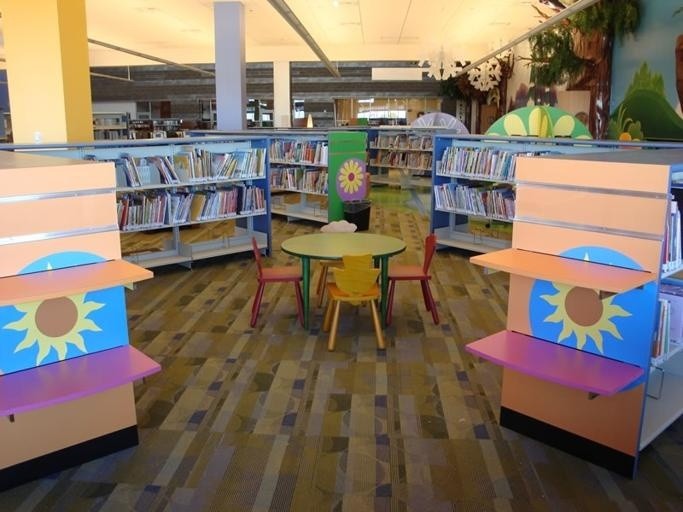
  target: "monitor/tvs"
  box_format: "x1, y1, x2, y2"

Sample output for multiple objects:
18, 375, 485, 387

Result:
651, 179, 683, 365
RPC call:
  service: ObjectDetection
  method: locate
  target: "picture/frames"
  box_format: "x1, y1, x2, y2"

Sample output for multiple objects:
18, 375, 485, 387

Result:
279, 231, 407, 332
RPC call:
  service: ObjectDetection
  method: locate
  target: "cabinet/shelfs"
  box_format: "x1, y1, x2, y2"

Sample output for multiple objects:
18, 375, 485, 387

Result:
270, 128, 683, 271
467, 149, 680, 478
2, 130, 274, 270
0, 150, 161, 489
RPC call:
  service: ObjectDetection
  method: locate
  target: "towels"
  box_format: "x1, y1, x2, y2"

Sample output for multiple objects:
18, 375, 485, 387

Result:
343, 199, 372, 232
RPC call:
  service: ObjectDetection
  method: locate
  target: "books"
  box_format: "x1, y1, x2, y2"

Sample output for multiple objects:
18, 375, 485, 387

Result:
270, 167, 328, 194
117, 183, 267, 230
95, 123, 187, 141
269, 140, 328, 165
651, 179, 683, 365
435, 183, 515, 220
369, 134, 432, 151
116, 146, 270, 187
368, 149, 433, 170
436, 146, 547, 179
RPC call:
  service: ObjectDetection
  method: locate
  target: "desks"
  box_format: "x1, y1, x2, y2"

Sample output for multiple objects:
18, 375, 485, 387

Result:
279, 231, 407, 332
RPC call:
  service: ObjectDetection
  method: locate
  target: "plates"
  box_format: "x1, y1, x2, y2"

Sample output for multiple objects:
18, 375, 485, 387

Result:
467, 149, 680, 478
0, 150, 161, 489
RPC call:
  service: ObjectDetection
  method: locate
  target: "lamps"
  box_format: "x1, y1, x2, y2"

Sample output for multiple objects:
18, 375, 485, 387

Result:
384, 233, 439, 326
323, 254, 387, 353
314, 219, 357, 308
247, 236, 307, 329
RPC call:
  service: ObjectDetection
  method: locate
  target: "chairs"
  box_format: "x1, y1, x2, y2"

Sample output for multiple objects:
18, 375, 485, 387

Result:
247, 236, 307, 329
314, 219, 357, 308
323, 254, 387, 353
384, 233, 439, 326
